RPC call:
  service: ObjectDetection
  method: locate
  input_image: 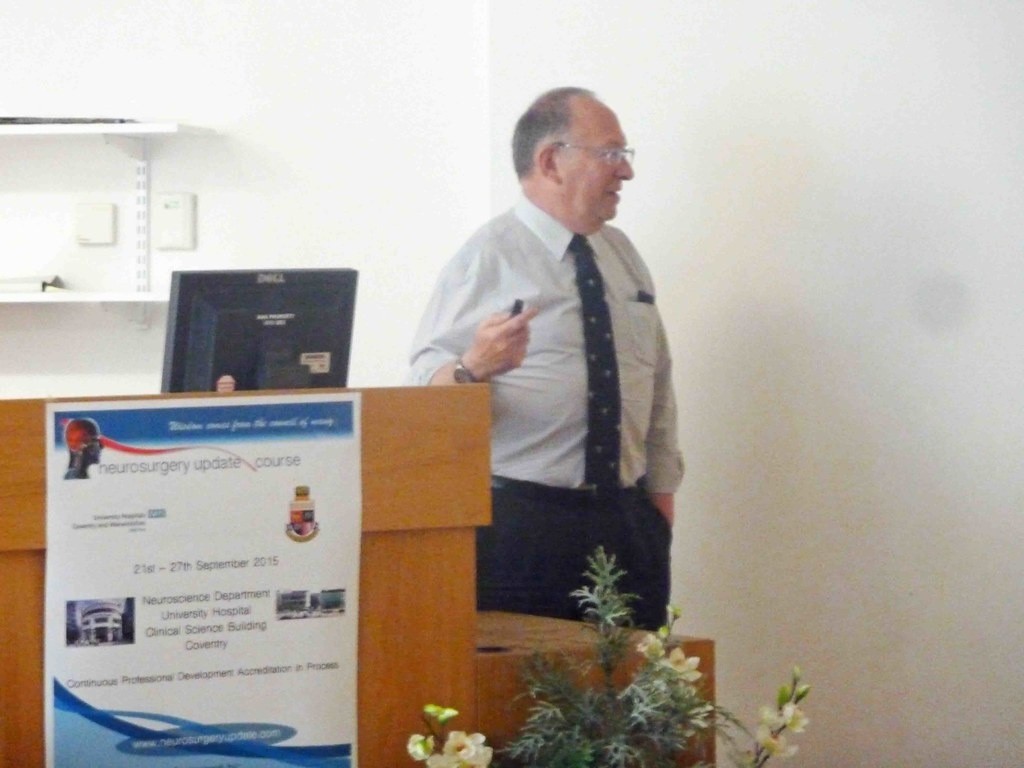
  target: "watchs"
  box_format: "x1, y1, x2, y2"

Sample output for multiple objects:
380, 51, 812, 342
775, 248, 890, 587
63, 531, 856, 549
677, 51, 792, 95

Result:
454, 359, 477, 383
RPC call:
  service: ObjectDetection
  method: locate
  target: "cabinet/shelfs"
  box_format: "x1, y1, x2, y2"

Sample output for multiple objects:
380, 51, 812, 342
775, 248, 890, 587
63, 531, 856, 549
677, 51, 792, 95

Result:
0, 120, 184, 312
474, 608, 717, 768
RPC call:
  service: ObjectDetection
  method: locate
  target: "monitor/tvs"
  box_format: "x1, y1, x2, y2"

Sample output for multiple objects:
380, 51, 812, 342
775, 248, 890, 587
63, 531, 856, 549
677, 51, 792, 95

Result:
159, 266, 358, 393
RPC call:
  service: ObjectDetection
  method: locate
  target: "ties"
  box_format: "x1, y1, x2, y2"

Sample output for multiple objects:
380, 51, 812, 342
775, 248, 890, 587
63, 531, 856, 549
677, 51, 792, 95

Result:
567, 232, 620, 489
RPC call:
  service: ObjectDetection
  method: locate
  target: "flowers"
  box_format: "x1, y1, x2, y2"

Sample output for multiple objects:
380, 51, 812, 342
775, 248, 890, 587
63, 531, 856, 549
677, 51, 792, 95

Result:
407, 544, 810, 768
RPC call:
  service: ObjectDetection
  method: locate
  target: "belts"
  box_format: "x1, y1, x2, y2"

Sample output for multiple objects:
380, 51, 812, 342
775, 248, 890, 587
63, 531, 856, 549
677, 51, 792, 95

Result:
489, 475, 650, 506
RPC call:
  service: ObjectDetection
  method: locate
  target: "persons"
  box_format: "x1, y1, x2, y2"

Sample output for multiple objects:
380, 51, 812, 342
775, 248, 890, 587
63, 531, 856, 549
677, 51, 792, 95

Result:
408, 86, 685, 633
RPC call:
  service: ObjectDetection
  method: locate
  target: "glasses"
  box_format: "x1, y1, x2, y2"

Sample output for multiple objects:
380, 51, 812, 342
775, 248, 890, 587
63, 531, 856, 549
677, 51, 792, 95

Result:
553, 142, 635, 166
